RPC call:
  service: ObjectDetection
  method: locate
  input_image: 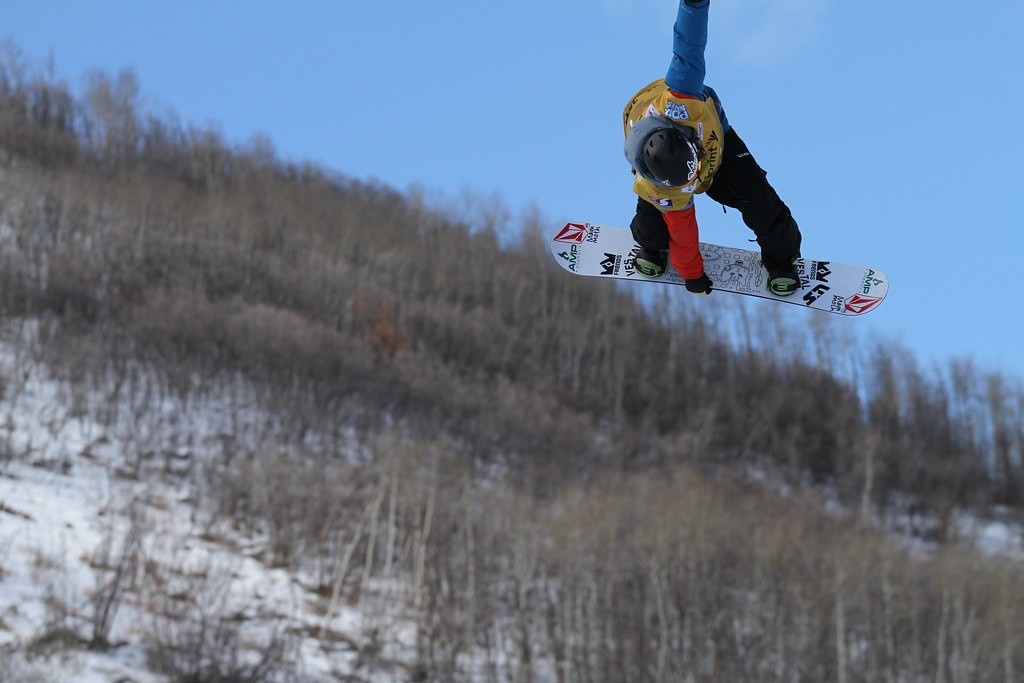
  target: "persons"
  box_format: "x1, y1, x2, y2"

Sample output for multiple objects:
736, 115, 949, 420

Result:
622, 0, 802, 296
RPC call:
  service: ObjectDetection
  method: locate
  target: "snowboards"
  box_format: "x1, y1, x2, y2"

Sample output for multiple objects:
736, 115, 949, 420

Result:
550, 222, 889, 316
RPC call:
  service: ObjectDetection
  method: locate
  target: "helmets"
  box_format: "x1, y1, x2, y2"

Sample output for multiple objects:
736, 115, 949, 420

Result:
642, 129, 697, 187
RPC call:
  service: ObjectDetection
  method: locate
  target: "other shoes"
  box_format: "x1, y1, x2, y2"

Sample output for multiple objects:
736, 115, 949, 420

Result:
632, 244, 668, 278
762, 253, 798, 297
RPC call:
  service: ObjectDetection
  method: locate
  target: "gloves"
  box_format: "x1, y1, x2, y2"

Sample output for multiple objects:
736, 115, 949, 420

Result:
685, 275, 714, 295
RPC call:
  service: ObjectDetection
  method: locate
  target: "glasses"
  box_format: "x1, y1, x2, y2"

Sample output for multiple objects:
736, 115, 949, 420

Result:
682, 161, 703, 189
